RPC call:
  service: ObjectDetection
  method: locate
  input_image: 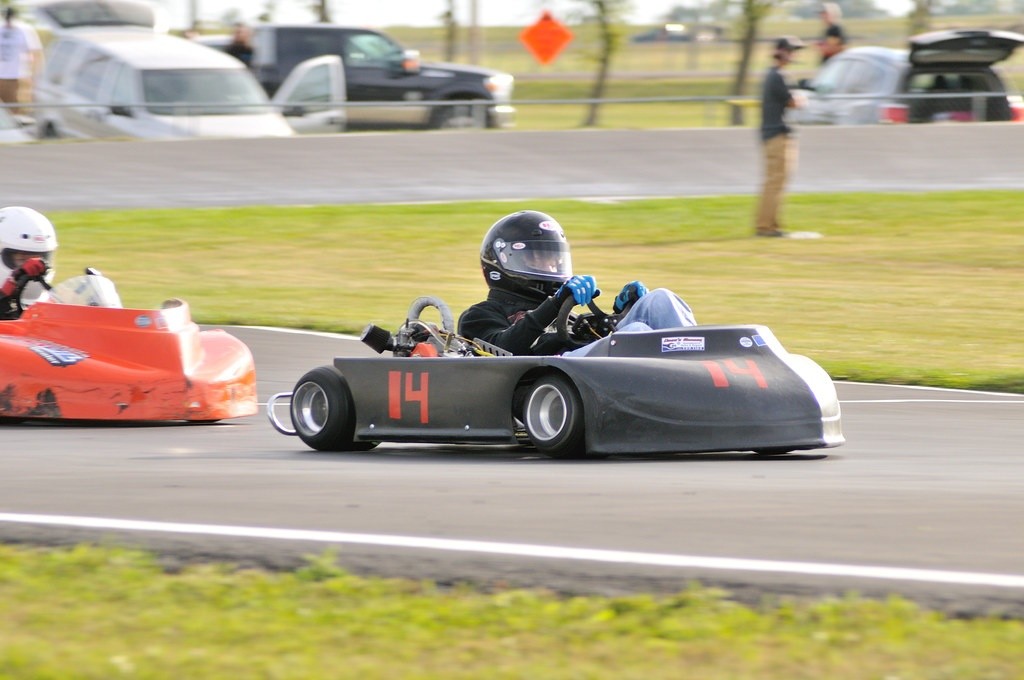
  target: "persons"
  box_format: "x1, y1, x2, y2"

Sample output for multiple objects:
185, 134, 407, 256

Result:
2, 204, 117, 333
808, 1, 853, 76
1, 5, 43, 114
746, 34, 811, 242
454, 210, 650, 361
221, 20, 256, 74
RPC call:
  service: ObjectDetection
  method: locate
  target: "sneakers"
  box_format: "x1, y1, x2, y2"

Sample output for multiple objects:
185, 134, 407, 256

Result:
757, 224, 787, 237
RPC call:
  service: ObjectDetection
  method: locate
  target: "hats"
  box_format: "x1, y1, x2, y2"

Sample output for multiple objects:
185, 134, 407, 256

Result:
775, 36, 805, 50
816, 3, 841, 14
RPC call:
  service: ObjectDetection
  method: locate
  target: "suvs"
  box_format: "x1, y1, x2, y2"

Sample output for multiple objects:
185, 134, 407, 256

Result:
235, 20, 518, 133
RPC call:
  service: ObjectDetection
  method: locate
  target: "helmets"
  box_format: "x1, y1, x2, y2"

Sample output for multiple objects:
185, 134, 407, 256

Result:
0, 206, 58, 289
480, 210, 573, 303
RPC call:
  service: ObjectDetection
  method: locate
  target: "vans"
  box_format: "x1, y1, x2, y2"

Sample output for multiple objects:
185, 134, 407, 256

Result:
25, 0, 349, 139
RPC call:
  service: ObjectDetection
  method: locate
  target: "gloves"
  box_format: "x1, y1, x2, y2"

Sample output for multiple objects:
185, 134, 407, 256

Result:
554, 275, 596, 306
9, 258, 49, 288
613, 281, 649, 315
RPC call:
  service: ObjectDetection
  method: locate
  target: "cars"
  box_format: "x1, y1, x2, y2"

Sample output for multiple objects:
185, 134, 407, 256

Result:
1, 100, 36, 145
635, 26, 714, 49
776, 27, 1024, 128
185, 31, 237, 56
